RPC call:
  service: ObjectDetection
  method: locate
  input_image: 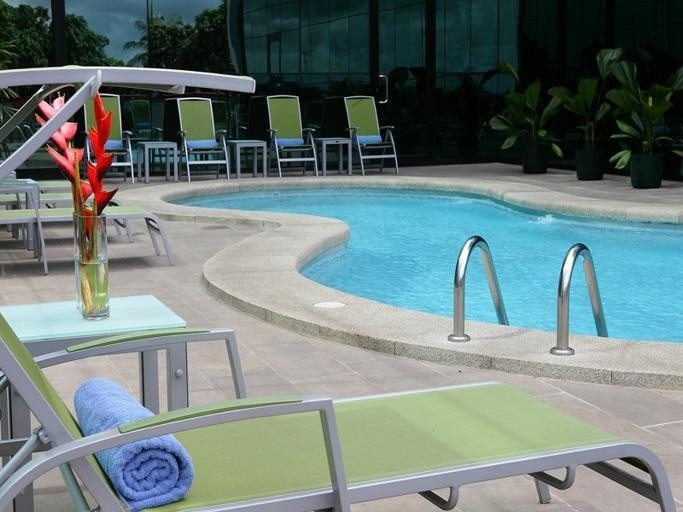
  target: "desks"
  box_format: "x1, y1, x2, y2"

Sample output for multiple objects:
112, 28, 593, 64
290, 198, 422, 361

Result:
315, 137, 352, 178
1, 294, 188, 511
137, 142, 176, 182
228, 140, 267, 178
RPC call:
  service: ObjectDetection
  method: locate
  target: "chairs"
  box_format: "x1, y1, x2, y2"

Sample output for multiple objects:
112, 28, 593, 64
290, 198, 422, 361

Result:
85, 93, 134, 185
0, 311, 678, 512
0, 159, 176, 277
177, 97, 229, 182
267, 95, 320, 178
343, 96, 398, 176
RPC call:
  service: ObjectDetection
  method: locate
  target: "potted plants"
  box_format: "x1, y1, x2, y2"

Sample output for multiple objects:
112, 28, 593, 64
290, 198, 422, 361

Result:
484, 47, 683, 188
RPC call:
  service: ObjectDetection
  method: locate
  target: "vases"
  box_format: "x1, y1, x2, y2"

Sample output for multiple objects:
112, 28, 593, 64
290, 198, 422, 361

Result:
71, 209, 112, 323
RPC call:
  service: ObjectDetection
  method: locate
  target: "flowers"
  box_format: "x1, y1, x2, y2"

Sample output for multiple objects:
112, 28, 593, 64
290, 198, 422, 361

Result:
33, 91, 120, 315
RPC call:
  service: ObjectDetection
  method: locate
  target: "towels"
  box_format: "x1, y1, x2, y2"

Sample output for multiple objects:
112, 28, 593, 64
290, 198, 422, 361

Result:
72, 376, 196, 512
